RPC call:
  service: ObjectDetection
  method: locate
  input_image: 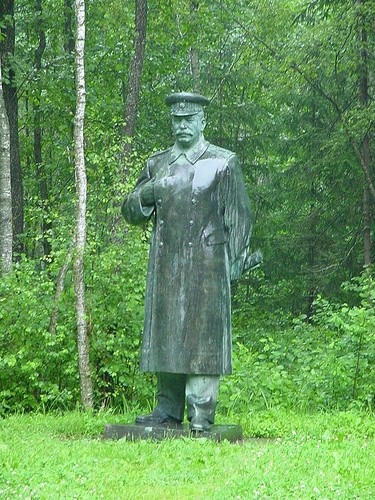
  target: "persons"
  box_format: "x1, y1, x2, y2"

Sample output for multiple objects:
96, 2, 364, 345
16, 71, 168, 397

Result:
119, 92, 256, 431
